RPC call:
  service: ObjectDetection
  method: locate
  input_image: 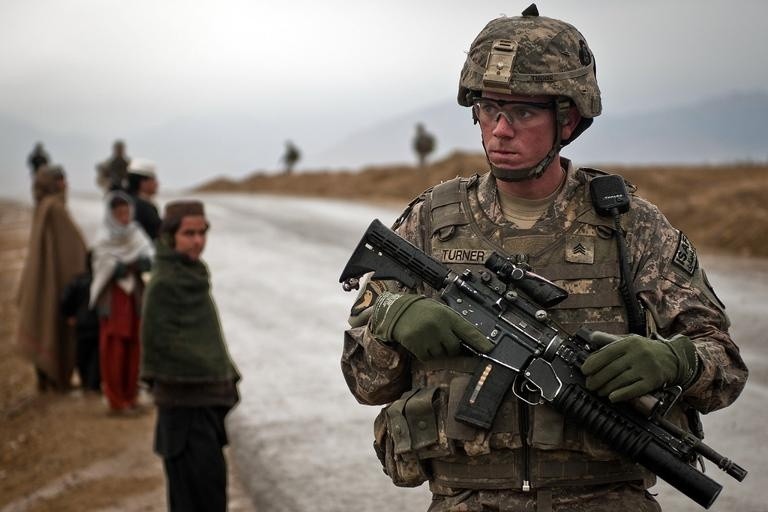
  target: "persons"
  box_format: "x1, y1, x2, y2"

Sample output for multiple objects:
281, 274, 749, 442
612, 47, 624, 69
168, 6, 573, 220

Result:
281, 140, 301, 175
11, 162, 89, 396
125, 170, 167, 242
337, 2, 753, 511
25, 138, 52, 171
136, 200, 244, 510
95, 138, 131, 195
413, 121, 437, 164
89, 190, 159, 417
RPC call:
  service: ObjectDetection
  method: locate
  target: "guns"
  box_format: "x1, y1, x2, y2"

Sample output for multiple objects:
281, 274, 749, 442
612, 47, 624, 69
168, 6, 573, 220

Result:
339, 219, 747, 509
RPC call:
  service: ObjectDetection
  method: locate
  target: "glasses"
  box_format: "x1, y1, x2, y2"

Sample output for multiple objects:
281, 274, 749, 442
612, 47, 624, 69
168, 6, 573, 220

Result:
470, 94, 558, 128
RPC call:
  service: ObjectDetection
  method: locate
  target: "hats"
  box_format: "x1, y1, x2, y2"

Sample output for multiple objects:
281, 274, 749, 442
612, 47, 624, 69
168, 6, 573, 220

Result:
164, 198, 204, 219
126, 158, 157, 178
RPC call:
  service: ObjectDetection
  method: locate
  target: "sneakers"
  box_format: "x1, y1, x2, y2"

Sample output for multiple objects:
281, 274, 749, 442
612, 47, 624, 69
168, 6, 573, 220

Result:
34, 378, 160, 419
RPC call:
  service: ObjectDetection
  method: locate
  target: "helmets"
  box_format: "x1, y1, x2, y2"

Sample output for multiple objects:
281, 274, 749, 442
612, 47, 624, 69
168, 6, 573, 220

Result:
457, 3, 602, 118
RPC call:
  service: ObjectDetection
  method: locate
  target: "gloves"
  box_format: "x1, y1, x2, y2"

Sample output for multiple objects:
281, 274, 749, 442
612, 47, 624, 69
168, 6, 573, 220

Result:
371, 291, 494, 363
580, 330, 699, 404
